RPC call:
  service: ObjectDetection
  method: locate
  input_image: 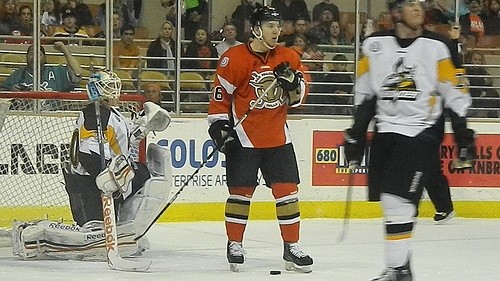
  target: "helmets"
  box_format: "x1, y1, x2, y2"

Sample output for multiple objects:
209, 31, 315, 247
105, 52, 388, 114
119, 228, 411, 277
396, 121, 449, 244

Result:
250, 6, 280, 21
87, 70, 122, 107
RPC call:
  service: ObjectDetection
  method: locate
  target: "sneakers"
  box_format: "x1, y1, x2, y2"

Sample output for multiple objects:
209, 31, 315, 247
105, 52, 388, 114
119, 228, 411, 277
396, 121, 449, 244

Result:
282, 242, 314, 272
226, 240, 246, 272
434, 209, 454, 222
372, 259, 412, 281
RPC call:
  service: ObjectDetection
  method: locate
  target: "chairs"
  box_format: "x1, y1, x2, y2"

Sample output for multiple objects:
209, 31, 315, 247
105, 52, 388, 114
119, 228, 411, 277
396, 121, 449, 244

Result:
0, 3, 500, 112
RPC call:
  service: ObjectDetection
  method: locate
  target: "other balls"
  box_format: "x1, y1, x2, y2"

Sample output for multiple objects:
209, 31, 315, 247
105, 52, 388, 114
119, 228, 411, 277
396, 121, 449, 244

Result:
270, 271, 281, 275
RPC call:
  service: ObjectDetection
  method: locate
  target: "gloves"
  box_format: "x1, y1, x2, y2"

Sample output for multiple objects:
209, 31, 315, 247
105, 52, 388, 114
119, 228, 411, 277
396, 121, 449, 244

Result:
209, 120, 240, 153
343, 129, 364, 170
274, 60, 299, 92
456, 128, 476, 168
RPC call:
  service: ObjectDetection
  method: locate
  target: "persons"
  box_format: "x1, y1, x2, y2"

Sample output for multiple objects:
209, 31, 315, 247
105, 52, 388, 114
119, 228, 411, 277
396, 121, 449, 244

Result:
11, 70, 172, 263
0, 41, 82, 111
146, 21, 184, 77
103, 23, 142, 87
343, 0, 478, 281
0, 0, 500, 224
49, 7, 92, 46
208, 6, 314, 266
314, 19, 352, 54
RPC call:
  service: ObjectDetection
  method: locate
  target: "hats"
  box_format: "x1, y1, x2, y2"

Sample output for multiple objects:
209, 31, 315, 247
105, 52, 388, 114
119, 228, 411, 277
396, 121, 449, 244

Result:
61, 10, 75, 19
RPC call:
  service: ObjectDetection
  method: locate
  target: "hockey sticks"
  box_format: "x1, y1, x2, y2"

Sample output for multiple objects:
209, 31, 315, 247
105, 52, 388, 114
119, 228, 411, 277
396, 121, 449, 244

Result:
132, 65, 291, 241
94, 101, 152, 271
336, 162, 356, 246
